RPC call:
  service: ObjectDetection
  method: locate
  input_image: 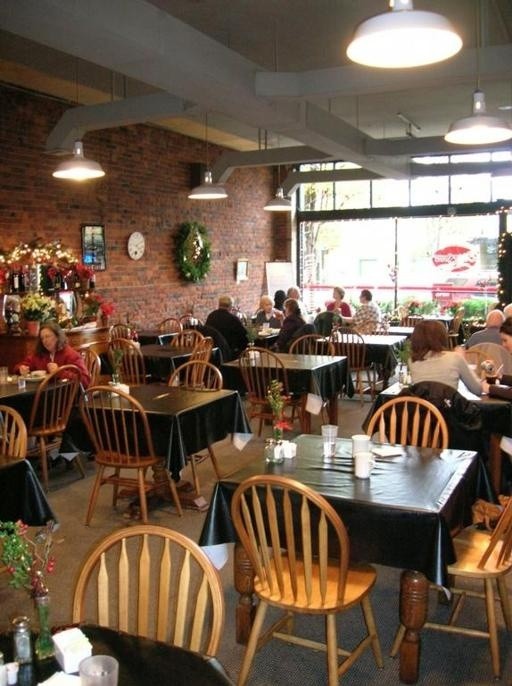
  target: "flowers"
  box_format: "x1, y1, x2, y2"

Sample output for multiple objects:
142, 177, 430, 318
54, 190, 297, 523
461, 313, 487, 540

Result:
99, 302, 114, 315
75, 262, 93, 279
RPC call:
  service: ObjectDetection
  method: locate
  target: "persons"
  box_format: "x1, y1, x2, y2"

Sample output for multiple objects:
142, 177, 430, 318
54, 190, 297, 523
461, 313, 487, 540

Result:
399, 298, 512, 404
202, 286, 381, 351
9, 322, 90, 471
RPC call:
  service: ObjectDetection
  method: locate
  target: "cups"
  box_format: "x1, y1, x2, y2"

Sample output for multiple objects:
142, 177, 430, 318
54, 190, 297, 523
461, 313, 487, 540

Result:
18, 380, 26, 388
351, 434, 376, 480
0, 367, 9, 385
262, 323, 269, 330
320, 424, 339, 458
0, 653, 19, 686
247, 350, 260, 361
79, 654, 120, 686
485, 374, 497, 385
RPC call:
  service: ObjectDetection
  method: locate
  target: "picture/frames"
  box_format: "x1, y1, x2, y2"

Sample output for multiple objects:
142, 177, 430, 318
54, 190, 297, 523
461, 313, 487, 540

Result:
235, 258, 249, 282
80, 223, 107, 272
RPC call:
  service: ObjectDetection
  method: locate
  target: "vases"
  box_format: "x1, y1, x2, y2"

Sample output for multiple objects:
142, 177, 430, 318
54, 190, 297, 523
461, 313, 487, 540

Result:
80, 278, 91, 291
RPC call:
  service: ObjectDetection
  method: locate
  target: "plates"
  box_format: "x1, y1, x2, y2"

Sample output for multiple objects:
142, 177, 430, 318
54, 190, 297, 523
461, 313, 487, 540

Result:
258, 332, 270, 336
19, 372, 46, 382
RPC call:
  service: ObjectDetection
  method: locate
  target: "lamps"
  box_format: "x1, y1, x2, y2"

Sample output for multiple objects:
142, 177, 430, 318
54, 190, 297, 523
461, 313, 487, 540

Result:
52, 57, 107, 180
187, 112, 228, 199
444, 0, 512, 145
346, 0, 463, 68
263, 165, 291, 211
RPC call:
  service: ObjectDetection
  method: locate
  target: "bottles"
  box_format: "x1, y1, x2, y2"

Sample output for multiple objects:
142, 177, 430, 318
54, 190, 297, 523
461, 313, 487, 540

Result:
10, 264, 26, 294
12, 615, 34, 666
263, 438, 284, 464
89, 266, 96, 291
398, 371, 412, 386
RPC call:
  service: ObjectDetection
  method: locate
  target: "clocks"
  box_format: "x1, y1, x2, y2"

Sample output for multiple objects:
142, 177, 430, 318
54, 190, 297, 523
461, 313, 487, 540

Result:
126, 232, 145, 261
